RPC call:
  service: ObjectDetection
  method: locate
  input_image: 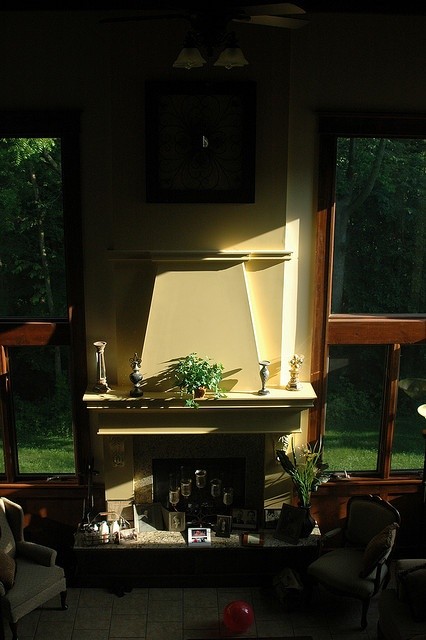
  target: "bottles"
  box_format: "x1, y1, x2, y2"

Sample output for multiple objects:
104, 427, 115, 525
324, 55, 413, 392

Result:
110, 520, 120, 533
100, 521, 109, 544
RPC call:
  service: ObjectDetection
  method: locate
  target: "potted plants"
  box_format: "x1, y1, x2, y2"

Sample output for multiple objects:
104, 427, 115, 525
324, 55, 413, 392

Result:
167, 352, 227, 410
277, 442, 325, 538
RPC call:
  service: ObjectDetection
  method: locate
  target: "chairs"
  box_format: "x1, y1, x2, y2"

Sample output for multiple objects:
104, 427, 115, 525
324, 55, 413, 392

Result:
0, 495, 69, 640
303, 493, 400, 627
